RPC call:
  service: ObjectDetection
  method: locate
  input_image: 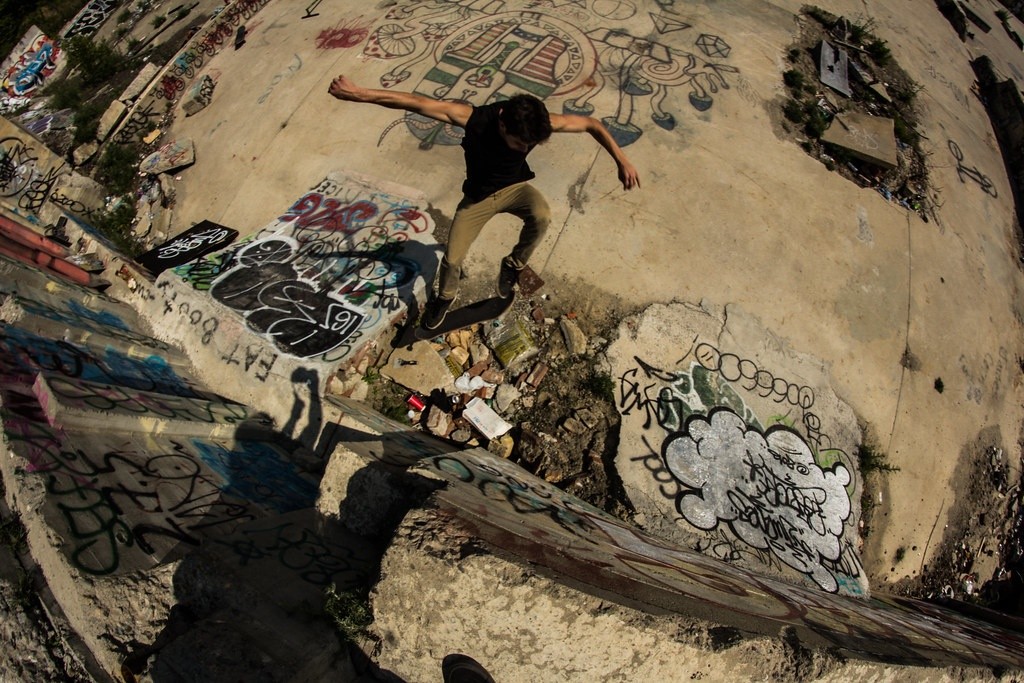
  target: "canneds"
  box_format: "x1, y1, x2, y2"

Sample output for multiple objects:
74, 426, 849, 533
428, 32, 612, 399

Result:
452, 395, 461, 403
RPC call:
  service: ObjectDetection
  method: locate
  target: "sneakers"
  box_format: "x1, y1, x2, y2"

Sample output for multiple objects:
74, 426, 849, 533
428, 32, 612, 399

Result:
495, 256, 523, 299
425, 294, 456, 329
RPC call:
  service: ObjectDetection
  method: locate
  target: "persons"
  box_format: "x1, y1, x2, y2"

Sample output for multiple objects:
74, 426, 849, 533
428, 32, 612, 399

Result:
327, 75, 643, 329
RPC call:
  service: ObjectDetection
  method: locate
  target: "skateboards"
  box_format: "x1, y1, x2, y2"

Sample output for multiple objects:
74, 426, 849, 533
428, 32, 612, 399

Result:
414, 289, 516, 342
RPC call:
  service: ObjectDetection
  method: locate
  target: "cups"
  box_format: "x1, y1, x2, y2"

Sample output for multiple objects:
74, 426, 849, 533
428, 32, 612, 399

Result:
408, 395, 426, 412
